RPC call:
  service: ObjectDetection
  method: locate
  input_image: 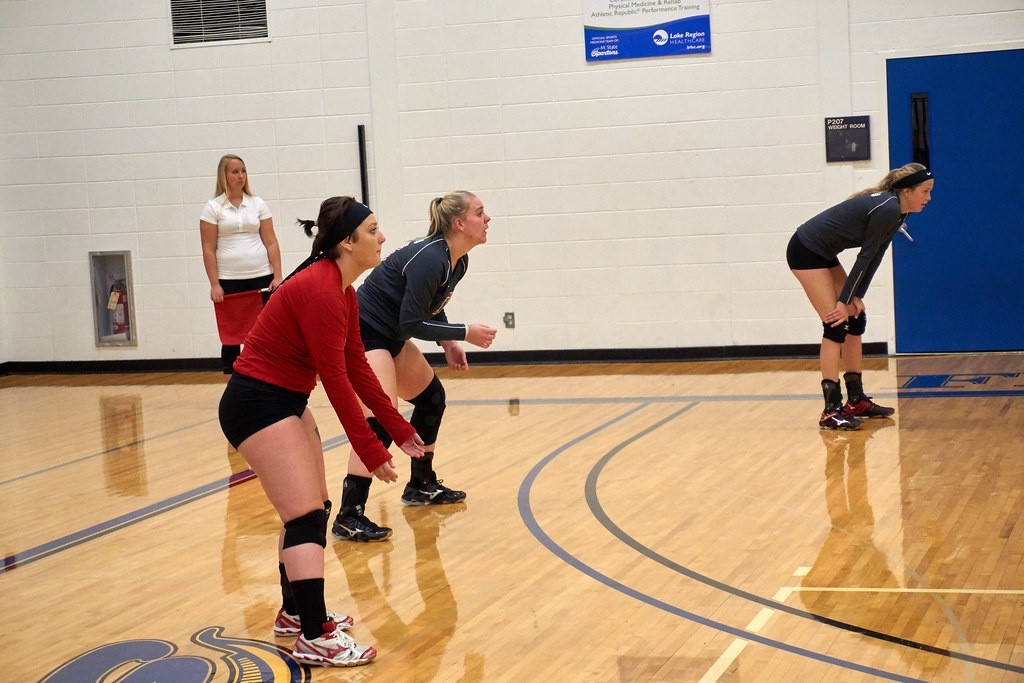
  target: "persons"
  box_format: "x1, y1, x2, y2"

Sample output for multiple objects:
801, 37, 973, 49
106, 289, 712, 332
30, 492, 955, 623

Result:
786, 163, 933, 430
332, 190, 497, 542
199, 153, 283, 384
222, 429, 285, 640
798, 418, 952, 676
219, 197, 426, 665
329, 500, 485, 683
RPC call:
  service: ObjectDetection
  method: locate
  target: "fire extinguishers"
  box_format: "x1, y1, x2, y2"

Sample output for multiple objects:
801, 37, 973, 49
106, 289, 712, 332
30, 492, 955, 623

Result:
107, 278, 130, 335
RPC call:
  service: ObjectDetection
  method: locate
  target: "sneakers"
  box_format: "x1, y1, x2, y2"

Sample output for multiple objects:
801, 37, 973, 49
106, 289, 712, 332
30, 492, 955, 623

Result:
844, 397, 895, 418
292, 621, 376, 667
274, 609, 353, 637
819, 406, 864, 431
331, 515, 393, 543
399, 472, 467, 504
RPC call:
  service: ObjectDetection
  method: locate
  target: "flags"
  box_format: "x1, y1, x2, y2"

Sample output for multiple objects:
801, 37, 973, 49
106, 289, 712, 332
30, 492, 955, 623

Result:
213, 289, 263, 345
